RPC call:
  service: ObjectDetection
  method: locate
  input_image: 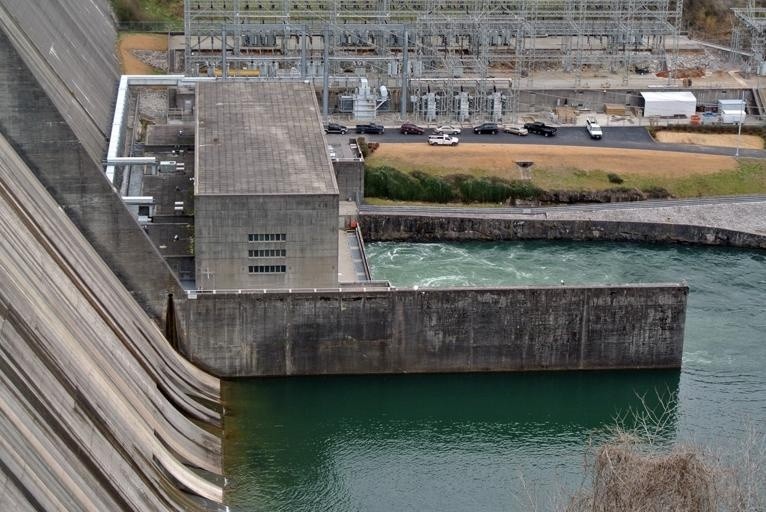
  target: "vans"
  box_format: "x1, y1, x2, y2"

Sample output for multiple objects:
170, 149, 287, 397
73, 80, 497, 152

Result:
586, 118, 603, 139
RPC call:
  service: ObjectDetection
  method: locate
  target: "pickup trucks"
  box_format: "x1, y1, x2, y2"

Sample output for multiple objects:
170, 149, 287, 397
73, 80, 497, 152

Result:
356, 122, 384, 135
524, 122, 557, 137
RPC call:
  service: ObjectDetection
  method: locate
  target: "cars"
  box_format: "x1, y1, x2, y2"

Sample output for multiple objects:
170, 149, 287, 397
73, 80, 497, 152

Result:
505, 126, 528, 136
433, 125, 460, 136
323, 122, 348, 135
427, 134, 459, 146
401, 124, 425, 135
472, 123, 498, 135
